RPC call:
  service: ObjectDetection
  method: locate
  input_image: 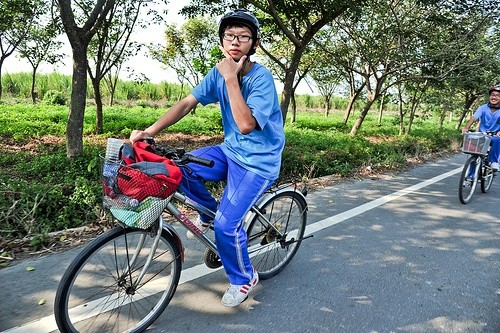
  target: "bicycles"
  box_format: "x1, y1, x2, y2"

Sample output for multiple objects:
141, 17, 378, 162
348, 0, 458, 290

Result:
53, 136, 314, 333
459, 129, 500, 205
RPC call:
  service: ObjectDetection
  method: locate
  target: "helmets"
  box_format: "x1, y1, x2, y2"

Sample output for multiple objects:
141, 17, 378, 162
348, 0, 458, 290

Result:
217, 10, 261, 54
490, 85, 500, 92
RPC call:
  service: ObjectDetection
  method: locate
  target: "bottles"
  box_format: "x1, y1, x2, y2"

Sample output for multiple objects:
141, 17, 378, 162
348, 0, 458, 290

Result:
102, 138, 125, 193
102, 194, 138, 209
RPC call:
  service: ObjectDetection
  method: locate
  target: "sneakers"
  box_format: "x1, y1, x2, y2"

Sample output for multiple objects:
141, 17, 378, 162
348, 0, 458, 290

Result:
221, 265, 259, 307
187, 215, 214, 240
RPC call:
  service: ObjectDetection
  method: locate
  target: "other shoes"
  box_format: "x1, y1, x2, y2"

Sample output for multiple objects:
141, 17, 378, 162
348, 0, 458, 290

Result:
491, 163, 499, 170
463, 178, 471, 186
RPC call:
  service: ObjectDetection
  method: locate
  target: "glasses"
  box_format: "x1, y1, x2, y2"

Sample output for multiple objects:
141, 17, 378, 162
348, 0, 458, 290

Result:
221, 33, 254, 42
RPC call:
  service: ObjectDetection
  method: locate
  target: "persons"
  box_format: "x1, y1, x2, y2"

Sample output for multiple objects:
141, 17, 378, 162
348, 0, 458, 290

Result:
131, 10, 286, 307
462, 86, 500, 181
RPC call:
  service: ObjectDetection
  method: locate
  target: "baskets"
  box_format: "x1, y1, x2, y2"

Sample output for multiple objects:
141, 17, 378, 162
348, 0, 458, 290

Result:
98, 152, 178, 232
462, 133, 492, 156
